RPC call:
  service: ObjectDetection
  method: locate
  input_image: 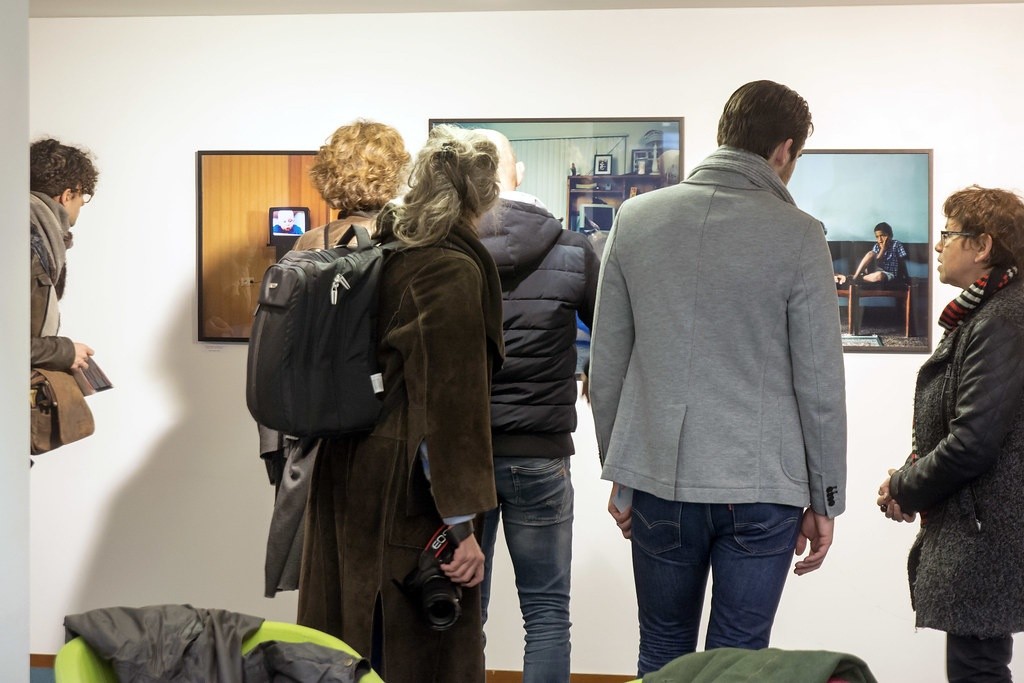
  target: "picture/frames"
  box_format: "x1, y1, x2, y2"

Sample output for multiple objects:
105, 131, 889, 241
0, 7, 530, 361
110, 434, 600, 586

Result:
786, 150, 934, 354
594, 155, 612, 175
197, 150, 343, 343
429, 116, 684, 381
632, 148, 665, 173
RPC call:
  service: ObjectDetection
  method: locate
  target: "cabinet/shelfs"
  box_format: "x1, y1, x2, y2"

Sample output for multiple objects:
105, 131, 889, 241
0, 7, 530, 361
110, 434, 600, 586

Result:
567, 175, 667, 230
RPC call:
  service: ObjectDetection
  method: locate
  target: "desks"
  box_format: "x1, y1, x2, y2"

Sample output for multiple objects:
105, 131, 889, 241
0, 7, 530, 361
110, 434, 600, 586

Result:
837, 285, 913, 339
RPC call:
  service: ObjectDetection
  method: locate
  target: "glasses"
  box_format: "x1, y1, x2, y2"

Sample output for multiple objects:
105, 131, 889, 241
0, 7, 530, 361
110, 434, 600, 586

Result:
68, 188, 93, 203
940, 230, 976, 245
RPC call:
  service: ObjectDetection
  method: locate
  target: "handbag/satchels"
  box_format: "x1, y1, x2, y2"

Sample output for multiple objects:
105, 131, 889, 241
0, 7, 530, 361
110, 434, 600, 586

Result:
29, 367, 94, 456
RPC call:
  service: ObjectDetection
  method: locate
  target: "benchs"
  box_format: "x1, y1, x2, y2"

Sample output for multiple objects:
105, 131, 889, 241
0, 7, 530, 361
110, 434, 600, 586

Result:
826, 242, 929, 338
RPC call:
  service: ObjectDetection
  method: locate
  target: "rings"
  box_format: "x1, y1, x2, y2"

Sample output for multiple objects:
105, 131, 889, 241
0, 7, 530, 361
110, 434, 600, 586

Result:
881, 505, 887, 512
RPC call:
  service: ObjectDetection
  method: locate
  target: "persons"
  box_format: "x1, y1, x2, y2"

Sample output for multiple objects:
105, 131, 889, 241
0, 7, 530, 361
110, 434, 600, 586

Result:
257, 119, 413, 600
273, 210, 303, 235
469, 130, 600, 683
848, 221, 913, 287
29, 137, 94, 373
297, 123, 505, 683
589, 80, 847, 678
877, 184, 1024, 683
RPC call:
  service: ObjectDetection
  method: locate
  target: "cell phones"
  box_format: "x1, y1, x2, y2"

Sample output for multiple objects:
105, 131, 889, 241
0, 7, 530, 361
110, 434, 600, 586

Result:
613, 486, 637, 513
880, 505, 915, 516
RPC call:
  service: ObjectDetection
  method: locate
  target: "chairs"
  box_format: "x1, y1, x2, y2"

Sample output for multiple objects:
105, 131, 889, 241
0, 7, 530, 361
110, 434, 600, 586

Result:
55, 621, 382, 683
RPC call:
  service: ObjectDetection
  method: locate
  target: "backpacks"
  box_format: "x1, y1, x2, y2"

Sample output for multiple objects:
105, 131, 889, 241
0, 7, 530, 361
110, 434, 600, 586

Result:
246, 224, 472, 434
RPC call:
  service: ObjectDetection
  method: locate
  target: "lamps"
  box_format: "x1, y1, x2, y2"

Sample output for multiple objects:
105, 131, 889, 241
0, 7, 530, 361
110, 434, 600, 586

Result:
645, 130, 663, 176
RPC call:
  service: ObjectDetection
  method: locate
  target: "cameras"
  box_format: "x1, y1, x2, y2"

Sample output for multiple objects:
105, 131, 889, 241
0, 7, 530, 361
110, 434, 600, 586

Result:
402, 556, 471, 632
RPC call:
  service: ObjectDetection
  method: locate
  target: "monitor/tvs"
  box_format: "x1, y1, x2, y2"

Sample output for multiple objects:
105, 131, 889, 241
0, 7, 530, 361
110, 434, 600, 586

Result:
580, 204, 615, 235
269, 207, 310, 244
